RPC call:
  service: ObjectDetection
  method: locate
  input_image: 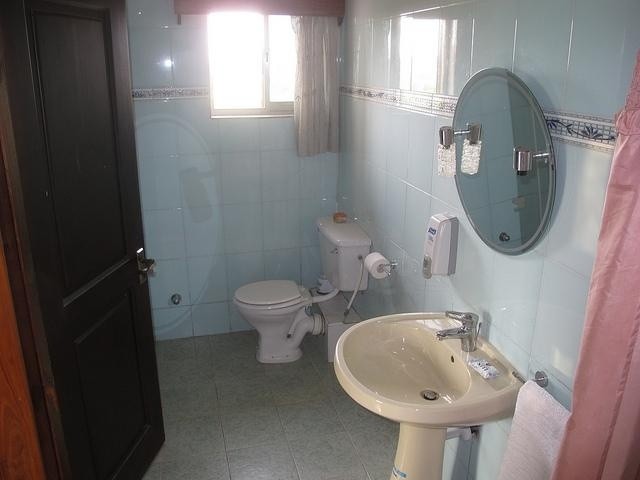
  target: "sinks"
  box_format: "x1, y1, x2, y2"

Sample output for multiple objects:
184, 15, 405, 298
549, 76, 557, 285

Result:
436, 310, 482, 352
334, 310, 524, 425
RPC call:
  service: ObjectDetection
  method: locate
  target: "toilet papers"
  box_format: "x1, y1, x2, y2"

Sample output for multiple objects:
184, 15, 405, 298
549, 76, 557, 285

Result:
364, 251, 390, 279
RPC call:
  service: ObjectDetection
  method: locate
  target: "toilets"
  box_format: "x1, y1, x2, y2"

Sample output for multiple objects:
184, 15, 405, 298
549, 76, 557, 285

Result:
233, 215, 373, 363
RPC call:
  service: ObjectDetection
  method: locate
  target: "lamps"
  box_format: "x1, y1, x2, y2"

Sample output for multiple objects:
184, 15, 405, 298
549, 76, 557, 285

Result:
512, 146, 551, 176
460, 120, 483, 177
437, 126, 461, 178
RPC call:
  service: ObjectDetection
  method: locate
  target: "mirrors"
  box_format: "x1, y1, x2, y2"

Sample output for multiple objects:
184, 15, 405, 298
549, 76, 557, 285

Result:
443, 67, 557, 257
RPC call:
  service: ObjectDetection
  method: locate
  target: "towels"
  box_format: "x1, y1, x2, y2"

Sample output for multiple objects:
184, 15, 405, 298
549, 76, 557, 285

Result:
500, 381, 574, 479
